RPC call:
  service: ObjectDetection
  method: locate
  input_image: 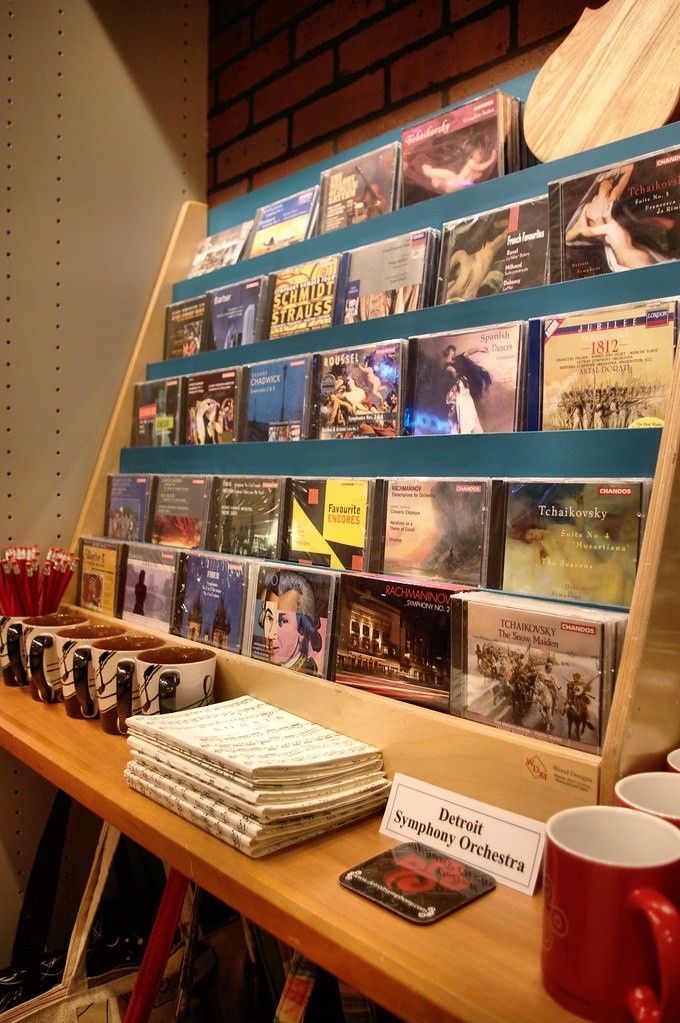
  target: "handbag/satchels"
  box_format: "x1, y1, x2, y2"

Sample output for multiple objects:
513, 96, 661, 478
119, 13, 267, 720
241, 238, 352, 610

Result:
0, 819, 287, 1023
0, 790, 240, 1013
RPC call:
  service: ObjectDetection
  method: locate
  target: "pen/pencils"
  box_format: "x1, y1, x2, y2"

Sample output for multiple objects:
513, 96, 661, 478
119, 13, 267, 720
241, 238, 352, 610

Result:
0, 545, 80, 617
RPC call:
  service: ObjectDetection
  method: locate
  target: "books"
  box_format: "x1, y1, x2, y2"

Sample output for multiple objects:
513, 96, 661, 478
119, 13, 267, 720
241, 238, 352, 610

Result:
124, 693, 392, 860
73, 474, 655, 757
162, 91, 680, 362
128, 296, 679, 447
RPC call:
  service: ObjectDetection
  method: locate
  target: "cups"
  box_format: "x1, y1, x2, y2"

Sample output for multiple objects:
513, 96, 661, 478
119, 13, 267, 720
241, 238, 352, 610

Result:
610, 771, 680, 829
54, 625, 128, 720
90, 635, 168, 736
0, 612, 58, 687
541, 804, 680, 1023
665, 748, 680, 772
22, 614, 90, 705
134, 645, 218, 716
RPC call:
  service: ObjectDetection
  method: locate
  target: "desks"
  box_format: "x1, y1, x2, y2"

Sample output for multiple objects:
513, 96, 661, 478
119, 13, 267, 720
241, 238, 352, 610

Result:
1, 673, 600, 1021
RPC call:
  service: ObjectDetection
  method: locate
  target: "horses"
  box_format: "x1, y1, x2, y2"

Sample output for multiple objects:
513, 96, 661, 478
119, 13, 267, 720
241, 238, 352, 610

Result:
474, 642, 590, 743
